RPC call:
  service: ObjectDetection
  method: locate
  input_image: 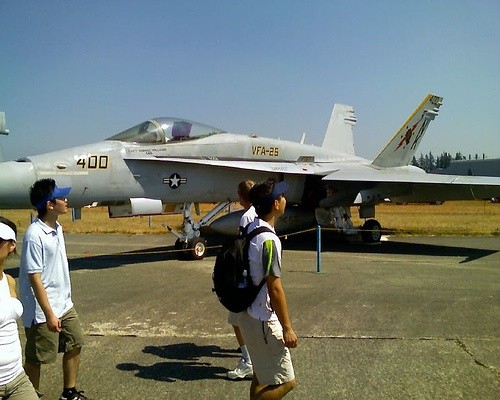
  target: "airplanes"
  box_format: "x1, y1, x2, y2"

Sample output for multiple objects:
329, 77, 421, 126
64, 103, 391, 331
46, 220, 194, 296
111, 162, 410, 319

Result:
0, 94, 500, 261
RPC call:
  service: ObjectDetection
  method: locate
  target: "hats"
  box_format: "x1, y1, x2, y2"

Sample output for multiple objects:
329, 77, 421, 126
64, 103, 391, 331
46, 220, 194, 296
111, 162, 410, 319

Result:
34, 179, 72, 206
0, 222, 17, 242
252, 178, 289, 215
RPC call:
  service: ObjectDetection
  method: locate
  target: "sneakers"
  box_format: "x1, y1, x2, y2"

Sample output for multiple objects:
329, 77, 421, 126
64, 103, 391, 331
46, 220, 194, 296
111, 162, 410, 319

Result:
227, 358, 254, 380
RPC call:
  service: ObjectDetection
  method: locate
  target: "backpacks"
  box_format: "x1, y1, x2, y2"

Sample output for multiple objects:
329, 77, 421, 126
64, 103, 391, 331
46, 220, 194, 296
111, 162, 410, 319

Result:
212, 223, 283, 313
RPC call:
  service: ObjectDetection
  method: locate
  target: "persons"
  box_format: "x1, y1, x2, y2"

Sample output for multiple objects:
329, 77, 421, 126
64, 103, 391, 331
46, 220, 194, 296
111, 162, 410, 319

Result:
15, 178, 91, 400
243, 183, 298, 400
228, 179, 257, 380
0, 215, 42, 400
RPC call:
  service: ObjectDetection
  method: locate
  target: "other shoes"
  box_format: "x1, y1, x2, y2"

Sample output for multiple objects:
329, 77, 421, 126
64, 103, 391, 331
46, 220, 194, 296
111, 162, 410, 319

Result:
59, 388, 90, 400
